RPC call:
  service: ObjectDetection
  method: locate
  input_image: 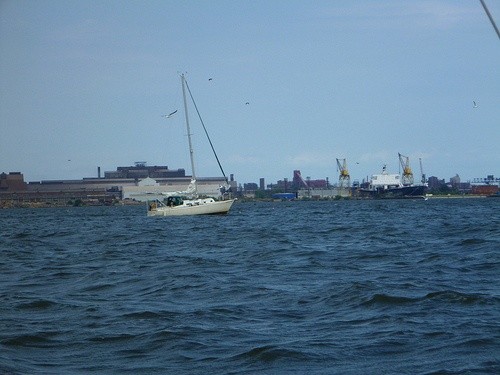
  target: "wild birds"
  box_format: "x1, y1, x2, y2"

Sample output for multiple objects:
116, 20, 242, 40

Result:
68, 160, 71, 161
473, 101, 478, 108
161, 110, 177, 119
246, 103, 249, 104
209, 78, 212, 81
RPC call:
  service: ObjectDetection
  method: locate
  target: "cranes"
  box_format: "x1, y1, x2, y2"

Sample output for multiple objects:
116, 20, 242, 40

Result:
418, 155, 430, 189
335, 156, 350, 189
398, 150, 414, 189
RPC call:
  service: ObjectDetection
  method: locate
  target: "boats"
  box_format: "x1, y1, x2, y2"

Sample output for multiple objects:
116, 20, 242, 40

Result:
144, 179, 232, 217
